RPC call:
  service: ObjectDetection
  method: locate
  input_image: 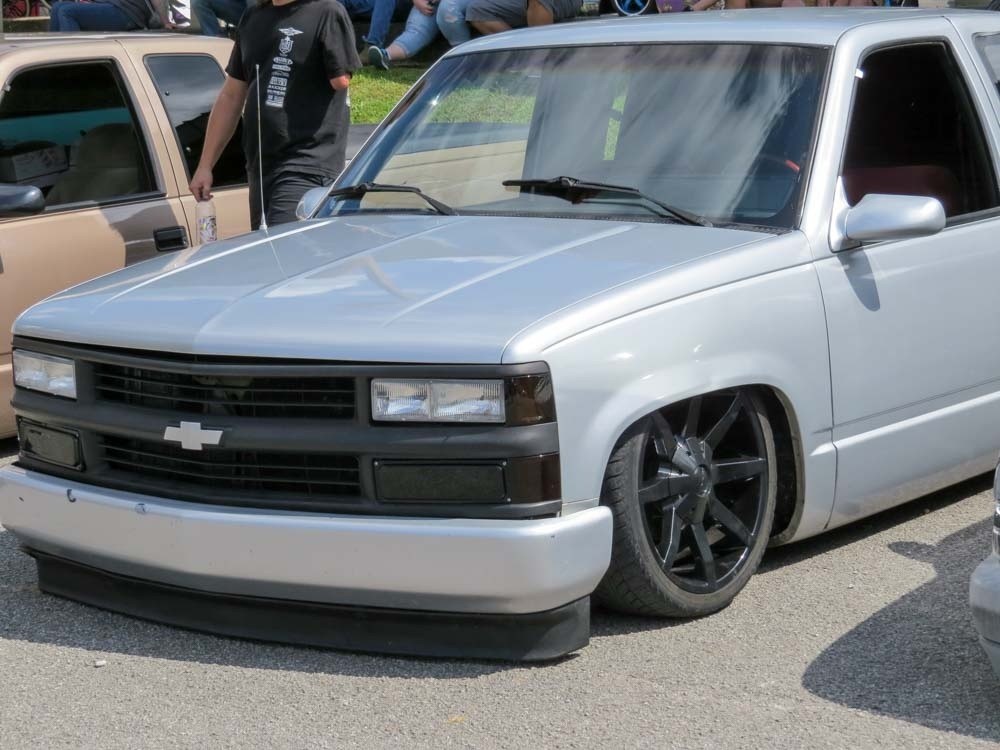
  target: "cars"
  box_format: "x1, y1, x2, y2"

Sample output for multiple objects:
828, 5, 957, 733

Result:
3, 9, 999, 648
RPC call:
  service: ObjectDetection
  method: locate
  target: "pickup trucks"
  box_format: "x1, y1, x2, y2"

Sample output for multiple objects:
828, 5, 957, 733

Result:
1, 34, 533, 440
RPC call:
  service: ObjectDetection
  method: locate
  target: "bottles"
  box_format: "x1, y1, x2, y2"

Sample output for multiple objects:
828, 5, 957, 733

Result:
196, 192, 217, 245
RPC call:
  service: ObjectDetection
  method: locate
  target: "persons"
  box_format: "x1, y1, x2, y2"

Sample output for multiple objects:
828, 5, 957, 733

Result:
337, 0, 479, 71
50, 0, 192, 34
464, 0, 584, 77
189, 0, 362, 229
597, 0, 921, 19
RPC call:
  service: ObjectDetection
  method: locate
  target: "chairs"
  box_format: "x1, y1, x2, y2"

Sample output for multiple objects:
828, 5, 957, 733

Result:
43, 123, 150, 204
842, 97, 961, 217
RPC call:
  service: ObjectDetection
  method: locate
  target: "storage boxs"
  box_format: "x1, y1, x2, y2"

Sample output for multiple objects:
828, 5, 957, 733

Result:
0, 138, 67, 191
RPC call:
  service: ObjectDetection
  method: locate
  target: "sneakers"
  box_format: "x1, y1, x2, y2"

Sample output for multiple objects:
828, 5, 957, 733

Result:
368, 45, 392, 71
